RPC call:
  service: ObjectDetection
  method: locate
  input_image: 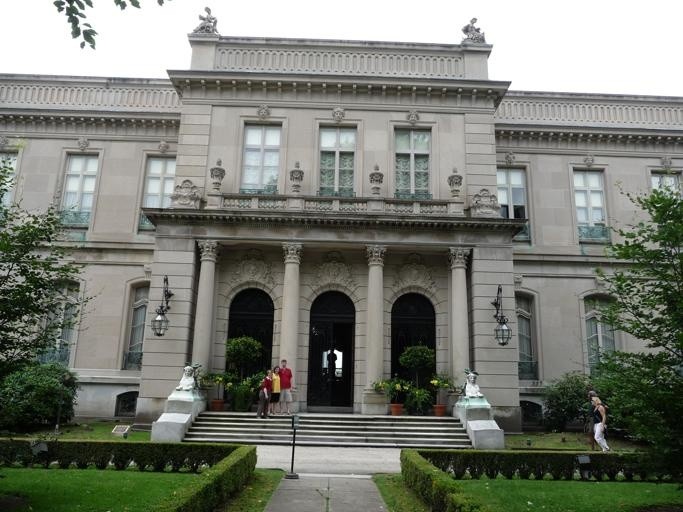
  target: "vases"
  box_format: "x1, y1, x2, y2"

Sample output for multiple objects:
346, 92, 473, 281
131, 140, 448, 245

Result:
433, 403, 446, 415
389, 401, 404, 415
210, 399, 224, 411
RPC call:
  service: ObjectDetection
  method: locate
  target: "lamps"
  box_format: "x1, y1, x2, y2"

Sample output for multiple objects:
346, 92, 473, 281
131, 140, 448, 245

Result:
151, 275, 174, 338
489, 283, 512, 348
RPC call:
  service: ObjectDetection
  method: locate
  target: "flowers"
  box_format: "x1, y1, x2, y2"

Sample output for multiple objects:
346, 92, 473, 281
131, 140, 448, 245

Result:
372, 374, 417, 403
430, 371, 464, 405
200, 369, 234, 400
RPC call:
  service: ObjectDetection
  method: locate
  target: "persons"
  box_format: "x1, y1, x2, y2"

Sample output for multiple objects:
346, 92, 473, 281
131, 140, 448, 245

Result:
326, 349, 336, 376
585, 389, 598, 450
255, 370, 272, 419
590, 397, 609, 450
465, 373, 480, 397
277, 359, 295, 415
270, 365, 280, 415
